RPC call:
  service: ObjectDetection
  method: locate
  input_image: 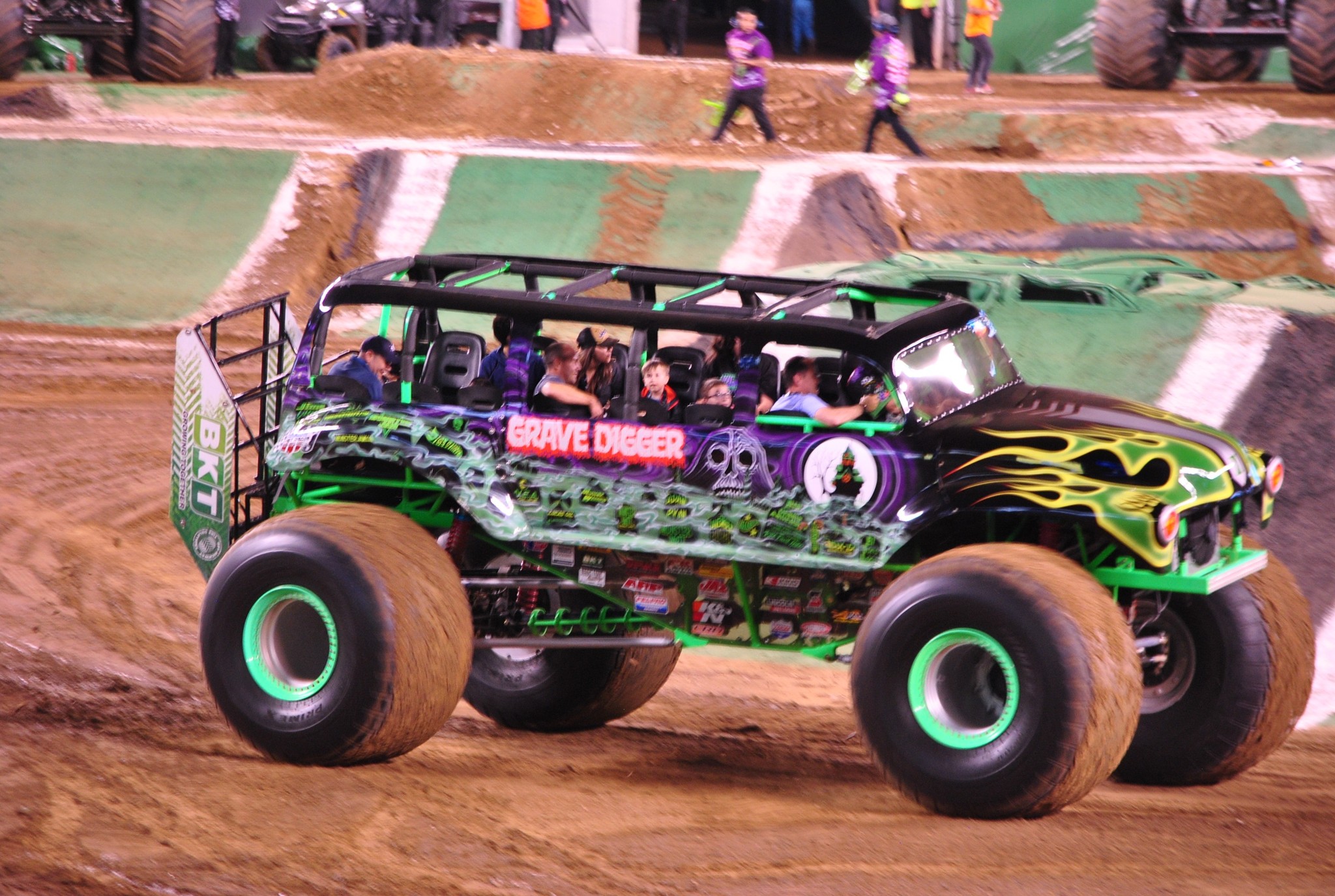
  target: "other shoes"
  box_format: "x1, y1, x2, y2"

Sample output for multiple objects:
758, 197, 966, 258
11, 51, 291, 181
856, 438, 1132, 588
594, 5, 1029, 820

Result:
974, 83, 993, 95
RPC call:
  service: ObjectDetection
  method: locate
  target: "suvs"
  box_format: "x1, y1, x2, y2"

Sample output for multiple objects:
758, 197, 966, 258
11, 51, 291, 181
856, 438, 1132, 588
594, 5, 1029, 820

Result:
166, 252, 1317, 820
1091, 0, 1335, 94
0, 0, 220, 81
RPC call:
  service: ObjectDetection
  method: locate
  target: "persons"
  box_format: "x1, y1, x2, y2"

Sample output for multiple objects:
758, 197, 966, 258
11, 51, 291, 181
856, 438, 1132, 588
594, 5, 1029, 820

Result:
514, 0, 1001, 157
327, 314, 973, 428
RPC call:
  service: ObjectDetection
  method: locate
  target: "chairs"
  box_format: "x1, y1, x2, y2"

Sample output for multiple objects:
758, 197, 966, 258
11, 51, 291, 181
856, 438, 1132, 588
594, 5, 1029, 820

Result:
314, 331, 779, 410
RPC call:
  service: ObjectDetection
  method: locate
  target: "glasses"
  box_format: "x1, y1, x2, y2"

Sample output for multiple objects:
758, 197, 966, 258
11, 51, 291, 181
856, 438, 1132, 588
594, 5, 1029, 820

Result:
703, 390, 732, 401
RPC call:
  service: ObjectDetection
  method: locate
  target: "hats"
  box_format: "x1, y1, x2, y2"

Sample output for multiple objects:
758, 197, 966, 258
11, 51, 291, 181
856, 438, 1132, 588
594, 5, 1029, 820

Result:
577, 327, 620, 349
359, 335, 399, 365
